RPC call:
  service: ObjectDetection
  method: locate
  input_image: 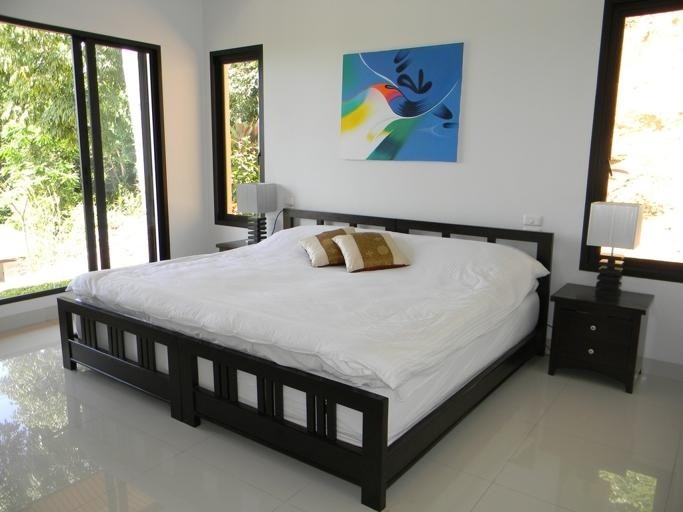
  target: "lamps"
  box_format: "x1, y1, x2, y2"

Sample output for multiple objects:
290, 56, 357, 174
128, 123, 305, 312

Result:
585, 201, 644, 301
237, 182, 278, 245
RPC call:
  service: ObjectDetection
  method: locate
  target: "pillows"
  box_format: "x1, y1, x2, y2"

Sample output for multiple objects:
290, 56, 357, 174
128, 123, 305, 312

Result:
330, 231, 411, 273
300, 225, 356, 266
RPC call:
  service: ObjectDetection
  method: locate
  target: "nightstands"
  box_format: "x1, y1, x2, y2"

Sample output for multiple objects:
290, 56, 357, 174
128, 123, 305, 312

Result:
548, 281, 655, 394
215, 238, 267, 251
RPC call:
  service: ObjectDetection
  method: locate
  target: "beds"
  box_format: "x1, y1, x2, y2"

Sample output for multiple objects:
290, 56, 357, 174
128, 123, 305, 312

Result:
54, 207, 556, 512
